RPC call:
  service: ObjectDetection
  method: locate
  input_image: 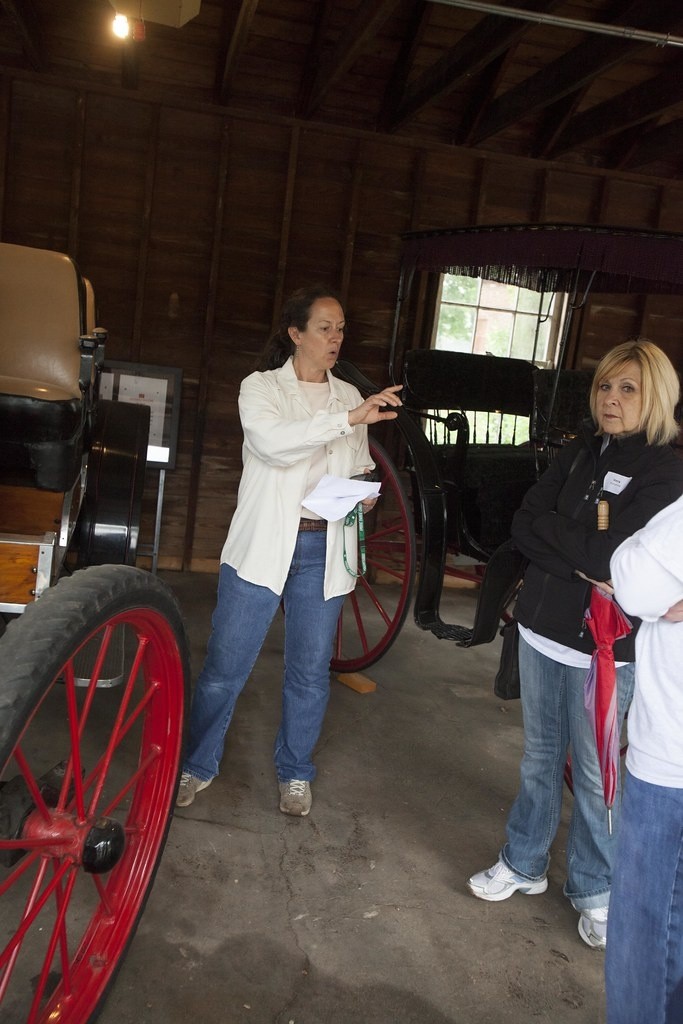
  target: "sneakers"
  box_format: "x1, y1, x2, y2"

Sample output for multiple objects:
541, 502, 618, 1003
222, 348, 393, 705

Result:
279, 779, 312, 816
175, 772, 212, 806
467, 859, 548, 901
571, 901, 609, 951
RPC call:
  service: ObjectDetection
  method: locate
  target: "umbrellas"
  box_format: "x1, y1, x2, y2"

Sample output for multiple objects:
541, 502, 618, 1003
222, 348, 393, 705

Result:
581, 496, 636, 836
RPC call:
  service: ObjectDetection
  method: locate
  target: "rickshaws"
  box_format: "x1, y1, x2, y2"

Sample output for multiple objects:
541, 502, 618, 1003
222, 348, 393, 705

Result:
1, 243, 193, 1024
279, 218, 682, 675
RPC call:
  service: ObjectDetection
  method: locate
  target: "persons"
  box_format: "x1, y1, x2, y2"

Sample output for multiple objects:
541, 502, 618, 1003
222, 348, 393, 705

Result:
464, 335, 683, 1023
170, 279, 407, 818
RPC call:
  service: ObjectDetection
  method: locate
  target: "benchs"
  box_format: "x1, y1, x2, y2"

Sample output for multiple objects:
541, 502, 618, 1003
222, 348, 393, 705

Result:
402, 344, 554, 489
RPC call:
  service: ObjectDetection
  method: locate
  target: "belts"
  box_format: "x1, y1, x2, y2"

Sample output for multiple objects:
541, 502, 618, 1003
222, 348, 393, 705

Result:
299, 518, 327, 531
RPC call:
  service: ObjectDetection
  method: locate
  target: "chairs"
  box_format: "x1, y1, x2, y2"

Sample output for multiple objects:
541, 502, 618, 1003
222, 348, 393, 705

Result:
0, 242, 113, 493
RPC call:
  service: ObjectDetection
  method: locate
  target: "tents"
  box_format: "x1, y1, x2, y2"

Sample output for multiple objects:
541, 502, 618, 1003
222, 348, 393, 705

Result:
386, 220, 683, 446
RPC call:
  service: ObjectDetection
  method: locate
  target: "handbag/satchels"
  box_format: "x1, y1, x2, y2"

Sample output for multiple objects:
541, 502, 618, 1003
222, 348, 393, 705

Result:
494, 618, 521, 700
